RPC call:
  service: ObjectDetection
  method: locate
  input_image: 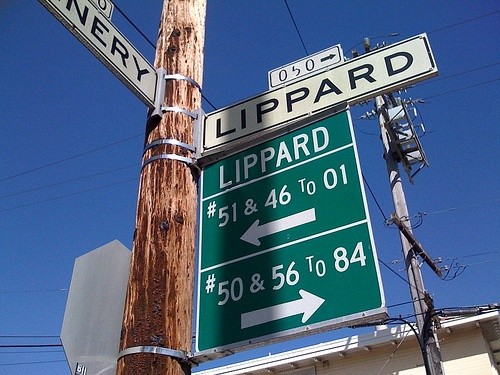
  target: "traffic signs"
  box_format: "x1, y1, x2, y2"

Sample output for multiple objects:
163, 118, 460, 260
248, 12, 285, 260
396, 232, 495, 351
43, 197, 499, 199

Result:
192, 109, 387, 352
